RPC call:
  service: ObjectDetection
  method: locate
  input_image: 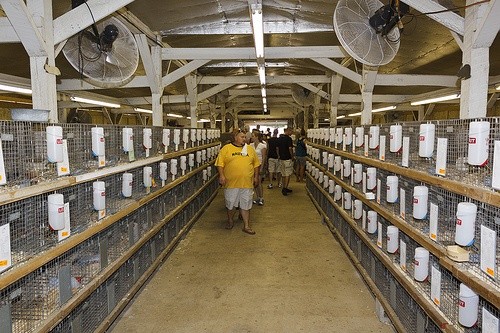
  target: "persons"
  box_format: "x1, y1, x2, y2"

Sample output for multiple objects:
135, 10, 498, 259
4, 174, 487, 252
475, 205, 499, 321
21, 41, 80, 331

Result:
244, 127, 307, 206
214, 128, 262, 235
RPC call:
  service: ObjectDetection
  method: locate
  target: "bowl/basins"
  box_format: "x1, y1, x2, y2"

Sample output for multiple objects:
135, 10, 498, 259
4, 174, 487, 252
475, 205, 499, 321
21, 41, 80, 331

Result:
446, 244, 469, 261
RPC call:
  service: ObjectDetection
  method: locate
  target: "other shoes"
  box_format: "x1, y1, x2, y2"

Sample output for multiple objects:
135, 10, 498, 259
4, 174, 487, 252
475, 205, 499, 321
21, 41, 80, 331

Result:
287, 188, 292, 193
300, 180, 306, 183
295, 180, 300, 182
243, 227, 255, 235
269, 184, 274, 188
259, 198, 264, 204
226, 220, 234, 228
277, 183, 281, 187
239, 214, 243, 220
282, 188, 287, 196
253, 198, 259, 203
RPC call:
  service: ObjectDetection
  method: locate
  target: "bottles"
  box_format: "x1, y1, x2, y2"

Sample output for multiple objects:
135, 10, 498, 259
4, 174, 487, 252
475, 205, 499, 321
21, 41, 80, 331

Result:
46, 126, 222, 231
305, 121, 493, 328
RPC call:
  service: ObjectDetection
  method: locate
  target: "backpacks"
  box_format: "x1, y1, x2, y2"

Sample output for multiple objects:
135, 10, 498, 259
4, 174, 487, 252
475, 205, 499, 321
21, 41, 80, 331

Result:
295, 138, 308, 157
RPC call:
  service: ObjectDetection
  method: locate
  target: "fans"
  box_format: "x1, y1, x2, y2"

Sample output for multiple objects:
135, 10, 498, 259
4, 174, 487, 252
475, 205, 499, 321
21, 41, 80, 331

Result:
62, 15, 139, 84
332, 0, 410, 67
215, 113, 232, 128
295, 111, 313, 129
203, 84, 229, 103
291, 83, 313, 107
